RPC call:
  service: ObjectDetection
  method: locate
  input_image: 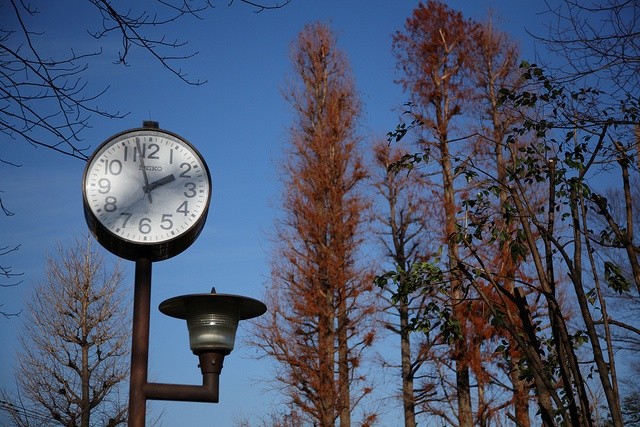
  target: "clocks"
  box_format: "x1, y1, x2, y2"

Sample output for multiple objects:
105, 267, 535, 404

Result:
82, 127, 212, 262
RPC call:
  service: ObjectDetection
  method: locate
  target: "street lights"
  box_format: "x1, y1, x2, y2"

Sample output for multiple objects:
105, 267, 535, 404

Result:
128, 287, 267, 427
